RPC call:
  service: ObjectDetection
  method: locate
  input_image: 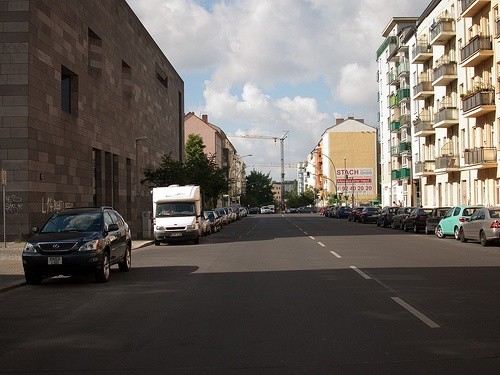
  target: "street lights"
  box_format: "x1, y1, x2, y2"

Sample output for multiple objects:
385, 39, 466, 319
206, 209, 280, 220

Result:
235, 165, 254, 202
228, 154, 252, 206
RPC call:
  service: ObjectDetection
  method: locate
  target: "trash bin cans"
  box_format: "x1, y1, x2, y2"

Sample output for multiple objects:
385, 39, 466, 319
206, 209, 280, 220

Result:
142, 211, 152, 239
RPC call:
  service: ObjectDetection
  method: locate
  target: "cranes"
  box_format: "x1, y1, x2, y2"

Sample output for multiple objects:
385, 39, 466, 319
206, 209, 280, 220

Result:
226, 130, 290, 213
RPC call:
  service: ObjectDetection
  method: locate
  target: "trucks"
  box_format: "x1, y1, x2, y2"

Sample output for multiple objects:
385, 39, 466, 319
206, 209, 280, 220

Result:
150, 185, 203, 246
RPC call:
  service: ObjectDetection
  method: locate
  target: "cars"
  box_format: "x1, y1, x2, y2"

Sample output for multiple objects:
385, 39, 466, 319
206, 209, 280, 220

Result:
424, 207, 453, 234
320, 205, 352, 218
434, 205, 484, 240
282, 206, 317, 214
360, 207, 380, 224
376, 206, 402, 228
390, 207, 417, 230
261, 206, 275, 214
403, 208, 435, 233
458, 207, 500, 247
205, 206, 247, 233
249, 207, 261, 214
203, 212, 212, 236
347, 208, 366, 222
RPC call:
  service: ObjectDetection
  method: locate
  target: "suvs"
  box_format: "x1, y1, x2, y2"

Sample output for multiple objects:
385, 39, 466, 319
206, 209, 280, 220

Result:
21, 207, 132, 285
